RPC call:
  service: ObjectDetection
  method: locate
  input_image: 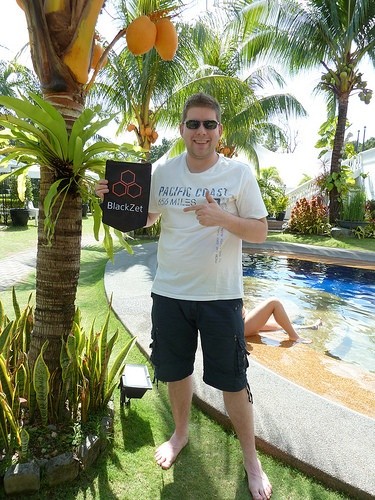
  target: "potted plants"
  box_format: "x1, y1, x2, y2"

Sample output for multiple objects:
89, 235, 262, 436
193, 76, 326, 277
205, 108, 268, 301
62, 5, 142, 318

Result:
7, 172, 34, 228
274, 196, 290, 222
335, 186, 370, 229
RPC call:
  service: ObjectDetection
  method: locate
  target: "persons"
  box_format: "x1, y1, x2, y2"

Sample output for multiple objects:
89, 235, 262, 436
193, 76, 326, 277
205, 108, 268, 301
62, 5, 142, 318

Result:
95, 93, 273, 500
242, 297, 322, 340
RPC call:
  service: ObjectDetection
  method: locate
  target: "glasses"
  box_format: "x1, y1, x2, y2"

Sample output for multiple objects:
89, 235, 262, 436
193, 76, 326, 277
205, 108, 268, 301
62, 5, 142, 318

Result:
183, 120, 220, 130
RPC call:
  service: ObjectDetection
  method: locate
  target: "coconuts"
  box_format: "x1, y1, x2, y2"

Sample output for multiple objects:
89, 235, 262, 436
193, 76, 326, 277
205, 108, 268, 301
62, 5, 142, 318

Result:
127, 125, 158, 140
320, 63, 372, 104
91, 44, 107, 70
219, 147, 231, 154
125, 15, 178, 61
